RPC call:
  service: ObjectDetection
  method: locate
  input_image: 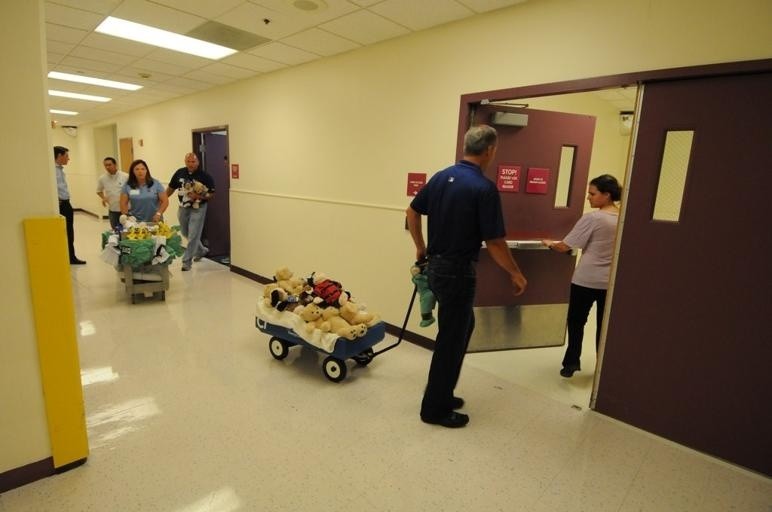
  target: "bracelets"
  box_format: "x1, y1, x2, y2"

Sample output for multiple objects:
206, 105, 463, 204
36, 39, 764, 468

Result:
156, 212, 161, 215
548, 243, 553, 249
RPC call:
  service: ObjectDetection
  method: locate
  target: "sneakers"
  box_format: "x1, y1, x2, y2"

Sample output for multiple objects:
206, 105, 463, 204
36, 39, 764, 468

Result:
70, 256, 87, 265
181, 249, 209, 271
449, 396, 465, 409
560, 364, 581, 378
420, 410, 470, 428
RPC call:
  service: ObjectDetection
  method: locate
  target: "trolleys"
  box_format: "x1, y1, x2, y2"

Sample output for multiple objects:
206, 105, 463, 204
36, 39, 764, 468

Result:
255, 257, 428, 382
117, 212, 171, 304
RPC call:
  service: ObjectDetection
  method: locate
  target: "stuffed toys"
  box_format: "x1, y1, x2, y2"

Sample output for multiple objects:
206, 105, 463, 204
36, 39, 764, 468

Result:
262, 268, 381, 341
119, 214, 170, 240
178, 180, 210, 209
412, 263, 436, 327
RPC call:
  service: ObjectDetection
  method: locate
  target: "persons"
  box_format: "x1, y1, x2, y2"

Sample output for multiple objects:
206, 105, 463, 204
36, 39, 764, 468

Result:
407, 123, 527, 428
95, 157, 131, 228
120, 159, 168, 223
54, 146, 86, 264
166, 153, 216, 271
542, 175, 622, 377
